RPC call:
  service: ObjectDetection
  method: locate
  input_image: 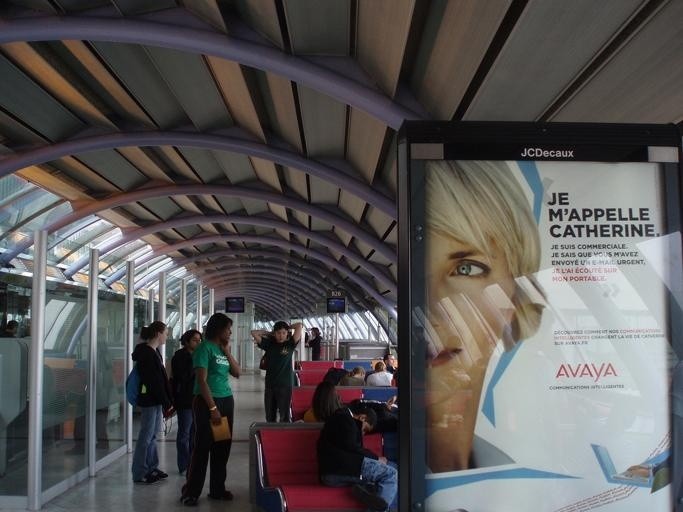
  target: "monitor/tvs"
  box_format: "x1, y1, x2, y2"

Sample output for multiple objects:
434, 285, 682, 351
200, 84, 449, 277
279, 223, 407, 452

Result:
326, 298, 345, 313
226, 297, 244, 313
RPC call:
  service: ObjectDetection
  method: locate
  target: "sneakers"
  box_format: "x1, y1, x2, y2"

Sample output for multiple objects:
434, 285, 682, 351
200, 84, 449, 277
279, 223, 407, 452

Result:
151, 469, 168, 478
133, 472, 160, 485
352, 478, 388, 511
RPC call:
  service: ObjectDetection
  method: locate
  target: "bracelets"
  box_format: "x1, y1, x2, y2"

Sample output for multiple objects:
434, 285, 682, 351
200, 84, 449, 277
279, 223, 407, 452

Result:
209, 405, 217, 411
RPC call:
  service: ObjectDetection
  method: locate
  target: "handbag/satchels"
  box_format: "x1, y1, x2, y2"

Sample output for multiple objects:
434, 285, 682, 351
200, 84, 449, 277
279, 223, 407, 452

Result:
126, 366, 142, 407
163, 377, 177, 418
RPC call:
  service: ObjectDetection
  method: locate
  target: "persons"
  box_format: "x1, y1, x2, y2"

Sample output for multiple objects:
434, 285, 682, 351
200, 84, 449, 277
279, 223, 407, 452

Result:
131, 321, 174, 485
251, 320, 302, 422
425, 159, 549, 478
0, 320, 18, 338
180, 313, 241, 506
306, 327, 323, 361
64, 326, 113, 455
170, 329, 201, 475
312, 354, 399, 512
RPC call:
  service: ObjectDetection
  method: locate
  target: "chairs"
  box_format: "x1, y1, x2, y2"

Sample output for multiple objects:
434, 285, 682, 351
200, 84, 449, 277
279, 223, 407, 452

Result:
39, 355, 127, 434
247, 359, 399, 510
